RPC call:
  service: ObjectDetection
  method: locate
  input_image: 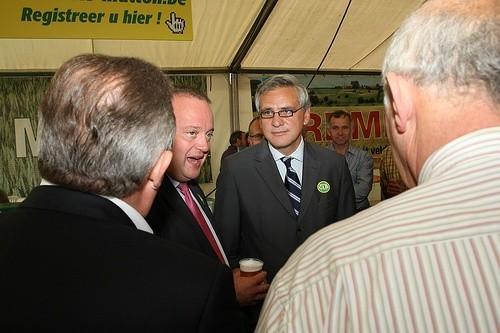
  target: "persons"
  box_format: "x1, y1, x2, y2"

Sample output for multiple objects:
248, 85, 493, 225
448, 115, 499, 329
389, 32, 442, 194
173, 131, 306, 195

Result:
325, 110, 374, 212
214, 74, 356, 283
0, 54, 240, 333
380, 146, 408, 200
254, 0, 500, 333
145, 86, 269, 333
220, 117, 264, 161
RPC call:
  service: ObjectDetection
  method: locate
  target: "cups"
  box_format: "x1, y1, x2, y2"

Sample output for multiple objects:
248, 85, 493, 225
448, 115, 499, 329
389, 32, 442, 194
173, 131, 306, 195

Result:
239, 258, 263, 277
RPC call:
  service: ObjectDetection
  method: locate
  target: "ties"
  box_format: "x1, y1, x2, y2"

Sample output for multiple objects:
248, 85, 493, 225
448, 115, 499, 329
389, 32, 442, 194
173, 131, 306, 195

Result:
280, 156, 301, 216
176, 181, 225, 264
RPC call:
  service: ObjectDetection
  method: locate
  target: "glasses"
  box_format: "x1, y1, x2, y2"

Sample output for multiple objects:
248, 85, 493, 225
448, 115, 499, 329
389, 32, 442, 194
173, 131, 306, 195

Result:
249, 133, 265, 140
258, 106, 303, 119
